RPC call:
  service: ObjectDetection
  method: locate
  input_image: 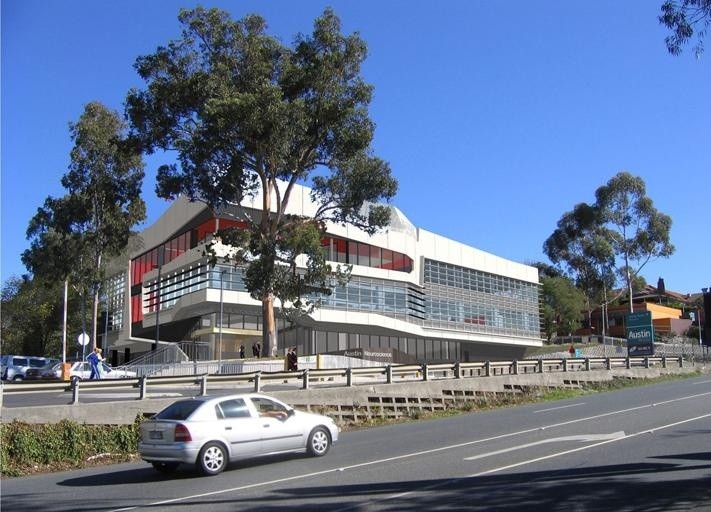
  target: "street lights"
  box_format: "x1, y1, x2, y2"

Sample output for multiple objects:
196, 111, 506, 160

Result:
152, 264, 163, 352
218, 270, 228, 374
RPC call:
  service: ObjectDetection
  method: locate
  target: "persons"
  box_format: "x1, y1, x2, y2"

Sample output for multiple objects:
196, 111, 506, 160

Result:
85, 347, 105, 380
94, 348, 106, 378
292, 348, 297, 371
239, 343, 245, 359
568, 345, 576, 358
287, 347, 297, 371
255, 340, 260, 358
252, 342, 257, 357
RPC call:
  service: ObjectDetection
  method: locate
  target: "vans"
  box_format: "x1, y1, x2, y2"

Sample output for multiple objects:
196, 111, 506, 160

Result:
0, 355, 63, 382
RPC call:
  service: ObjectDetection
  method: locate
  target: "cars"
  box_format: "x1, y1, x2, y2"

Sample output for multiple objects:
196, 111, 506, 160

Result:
138, 392, 339, 476
27, 361, 137, 382
